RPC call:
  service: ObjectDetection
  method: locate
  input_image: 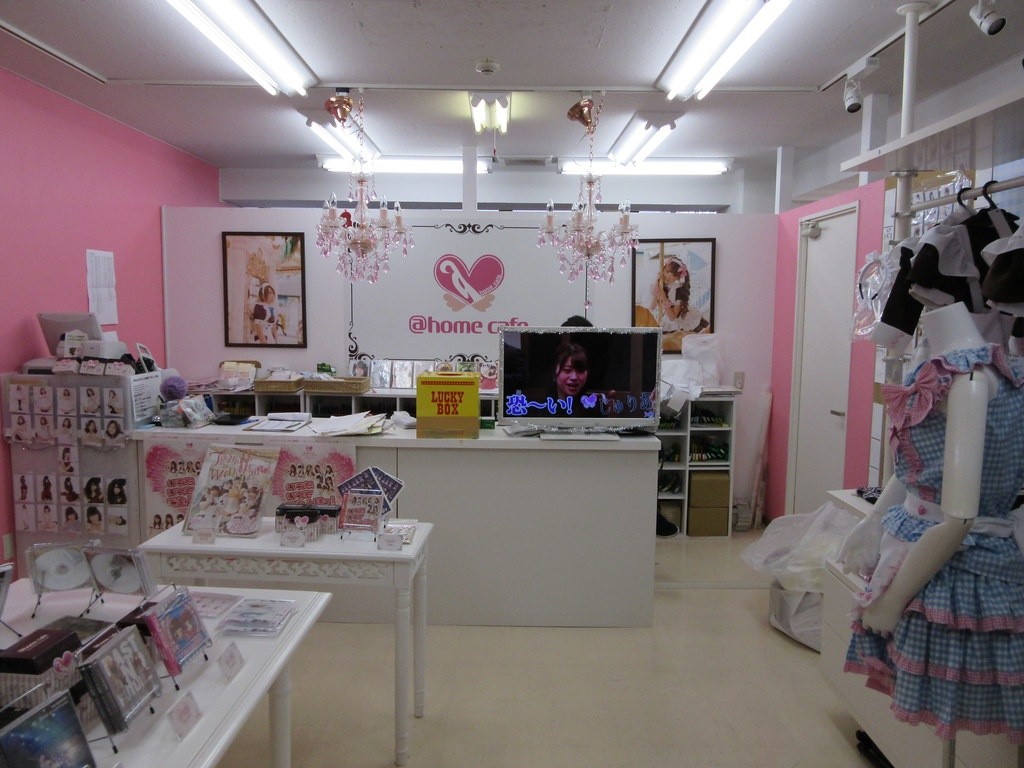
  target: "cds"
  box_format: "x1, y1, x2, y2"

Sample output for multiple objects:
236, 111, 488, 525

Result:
34, 550, 91, 591
92, 554, 140, 593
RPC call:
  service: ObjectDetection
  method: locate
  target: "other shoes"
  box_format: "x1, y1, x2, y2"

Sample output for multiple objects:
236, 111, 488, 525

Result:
656, 513, 679, 538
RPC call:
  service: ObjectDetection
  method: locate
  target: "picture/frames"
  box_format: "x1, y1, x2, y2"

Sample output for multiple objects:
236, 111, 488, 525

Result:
632, 238, 717, 354
222, 231, 307, 349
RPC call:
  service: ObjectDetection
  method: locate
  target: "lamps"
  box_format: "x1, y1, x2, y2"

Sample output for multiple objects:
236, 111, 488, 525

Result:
315, 154, 495, 176
654, 0, 793, 102
969, 0, 1008, 37
468, 91, 511, 136
607, 111, 685, 165
166, 0, 319, 98
296, 107, 381, 162
843, 78, 862, 113
537, 88, 640, 309
555, 156, 735, 176
315, 87, 415, 284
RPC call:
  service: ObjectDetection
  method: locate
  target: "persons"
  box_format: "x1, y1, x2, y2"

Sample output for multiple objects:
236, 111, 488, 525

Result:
352, 496, 379, 515
253, 284, 278, 343
170, 461, 200, 476
650, 256, 709, 334
149, 514, 184, 537
835, 300, 1024, 747
540, 343, 610, 418
561, 316, 594, 327
199, 475, 263, 536
352, 361, 368, 377
287, 463, 337, 499
10, 385, 127, 535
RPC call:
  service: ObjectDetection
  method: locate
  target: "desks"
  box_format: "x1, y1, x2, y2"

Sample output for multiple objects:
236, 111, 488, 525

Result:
138, 517, 434, 765
0, 576, 331, 768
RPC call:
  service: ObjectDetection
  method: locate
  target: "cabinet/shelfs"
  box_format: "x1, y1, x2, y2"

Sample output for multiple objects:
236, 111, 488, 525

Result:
191, 390, 498, 422
826, 488, 1024, 768
659, 393, 736, 540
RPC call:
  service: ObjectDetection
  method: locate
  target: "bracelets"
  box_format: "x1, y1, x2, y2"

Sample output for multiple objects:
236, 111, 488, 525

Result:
663, 300, 672, 308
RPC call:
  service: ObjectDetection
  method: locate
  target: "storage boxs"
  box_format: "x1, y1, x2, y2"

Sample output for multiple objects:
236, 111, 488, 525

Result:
661, 505, 680, 527
0, 626, 81, 675
688, 472, 729, 536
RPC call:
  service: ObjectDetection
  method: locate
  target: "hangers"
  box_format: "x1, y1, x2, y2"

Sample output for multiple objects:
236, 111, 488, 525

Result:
957, 180, 1021, 224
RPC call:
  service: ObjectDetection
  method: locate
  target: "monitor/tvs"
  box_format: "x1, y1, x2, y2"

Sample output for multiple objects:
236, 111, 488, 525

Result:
497, 325, 662, 440
37, 312, 105, 357
